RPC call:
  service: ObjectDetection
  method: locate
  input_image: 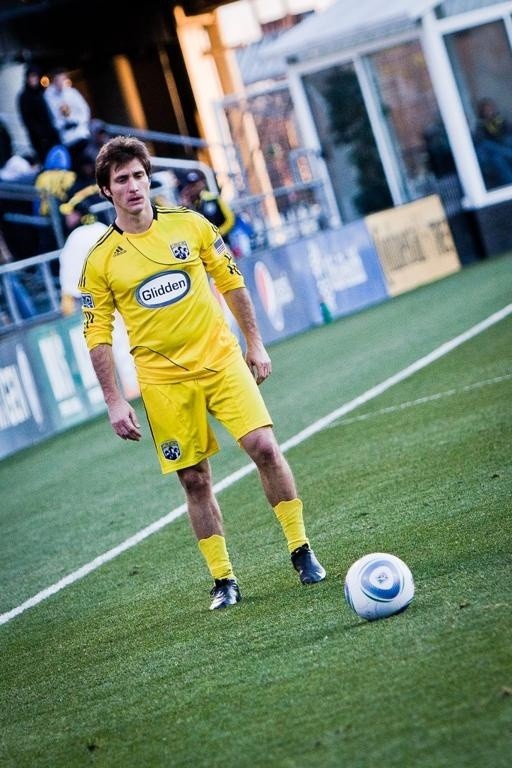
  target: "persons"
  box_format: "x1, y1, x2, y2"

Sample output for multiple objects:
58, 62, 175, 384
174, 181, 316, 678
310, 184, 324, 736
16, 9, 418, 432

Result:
59, 202, 142, 402
474, 99, 512, 147
421, 126, 512, 185
76, 134, 328, 616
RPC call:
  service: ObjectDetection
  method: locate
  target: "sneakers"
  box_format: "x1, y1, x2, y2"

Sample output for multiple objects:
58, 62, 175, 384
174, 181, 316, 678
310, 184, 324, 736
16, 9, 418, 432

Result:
209, 577, 241, 609
290, 543, 326, 584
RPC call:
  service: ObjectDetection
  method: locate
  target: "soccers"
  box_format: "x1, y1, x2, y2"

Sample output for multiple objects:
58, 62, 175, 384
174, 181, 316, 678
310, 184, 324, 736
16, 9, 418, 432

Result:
340, 550, 412, 621
